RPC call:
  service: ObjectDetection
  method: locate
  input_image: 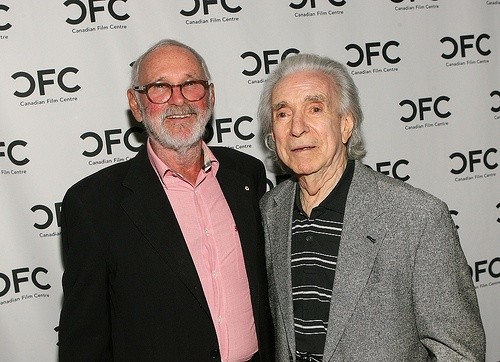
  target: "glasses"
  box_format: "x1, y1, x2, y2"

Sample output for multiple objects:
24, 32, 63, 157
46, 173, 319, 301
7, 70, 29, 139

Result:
135, 80, 209, 104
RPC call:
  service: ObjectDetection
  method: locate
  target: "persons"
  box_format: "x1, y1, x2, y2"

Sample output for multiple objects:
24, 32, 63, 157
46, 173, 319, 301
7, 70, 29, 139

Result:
260, 54, 486, 362
57, 39, 277, 362
